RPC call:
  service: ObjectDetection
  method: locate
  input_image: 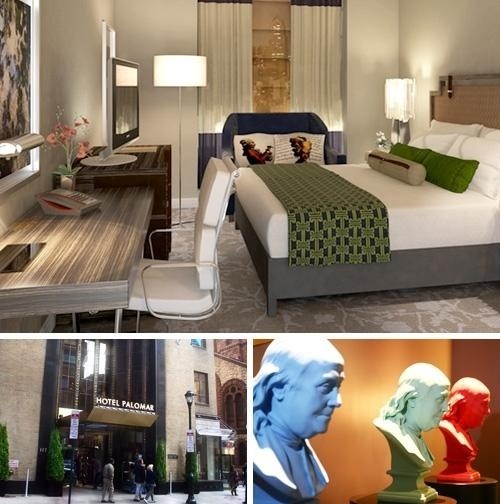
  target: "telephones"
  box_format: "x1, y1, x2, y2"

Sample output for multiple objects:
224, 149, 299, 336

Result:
37, 185, 103, 216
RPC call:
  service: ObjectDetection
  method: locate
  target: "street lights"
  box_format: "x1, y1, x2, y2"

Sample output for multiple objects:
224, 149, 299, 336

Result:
185, 390, 197, 504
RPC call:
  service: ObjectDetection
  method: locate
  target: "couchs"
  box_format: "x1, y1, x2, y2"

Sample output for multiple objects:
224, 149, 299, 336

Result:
222, 112, 346, 220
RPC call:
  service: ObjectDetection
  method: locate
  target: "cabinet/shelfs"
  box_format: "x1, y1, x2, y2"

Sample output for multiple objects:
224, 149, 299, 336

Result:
53, 143, 172, 261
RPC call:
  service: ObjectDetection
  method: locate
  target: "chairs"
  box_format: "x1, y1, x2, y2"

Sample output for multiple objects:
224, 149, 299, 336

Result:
124, 157, 238, 335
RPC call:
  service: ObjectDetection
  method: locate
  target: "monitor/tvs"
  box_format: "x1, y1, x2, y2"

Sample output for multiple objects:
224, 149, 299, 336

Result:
78, 56, 140, 165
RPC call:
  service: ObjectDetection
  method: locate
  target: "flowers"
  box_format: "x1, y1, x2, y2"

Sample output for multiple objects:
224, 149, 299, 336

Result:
45, 104, 91, 176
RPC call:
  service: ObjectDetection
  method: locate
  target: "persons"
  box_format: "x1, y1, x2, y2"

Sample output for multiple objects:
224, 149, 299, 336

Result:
252, 339, 345, 499
242, 467, 247, 504
228, 465, 239, 495
143, 464, 157, 503
134, 460, 146, 501
101, 457, 115, 503
372, 363, 451, 503
91, 456, 103, 490
437, 377, 490, 482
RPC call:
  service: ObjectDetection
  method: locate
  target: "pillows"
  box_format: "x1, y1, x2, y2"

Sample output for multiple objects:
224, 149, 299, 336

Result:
384, 141, 434, 167
427, 117, 485, 143
477, 125, 500, 147
445, 132, 500, 200
231, 131, 280, 167
274, 131, 325, 166
412, 132, 471, 158
418, 151, 480, 195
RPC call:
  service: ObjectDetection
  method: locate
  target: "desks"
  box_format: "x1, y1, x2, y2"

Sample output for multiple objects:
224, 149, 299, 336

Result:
0, 185, 155, 335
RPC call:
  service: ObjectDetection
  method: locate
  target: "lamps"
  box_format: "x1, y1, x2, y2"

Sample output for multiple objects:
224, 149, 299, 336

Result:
0, 132, 51, 159
151, 54, 209, 230
383, 77, 417, 149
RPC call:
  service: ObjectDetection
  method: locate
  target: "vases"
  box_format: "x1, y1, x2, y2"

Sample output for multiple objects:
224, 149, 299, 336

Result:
55, 172, 79, 194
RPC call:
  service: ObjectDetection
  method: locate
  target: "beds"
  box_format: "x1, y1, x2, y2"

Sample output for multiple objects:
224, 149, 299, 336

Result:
231, 75, 500, 318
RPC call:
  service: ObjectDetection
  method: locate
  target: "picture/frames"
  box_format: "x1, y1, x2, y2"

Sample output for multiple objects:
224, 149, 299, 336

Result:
0, 0, 43, 203
